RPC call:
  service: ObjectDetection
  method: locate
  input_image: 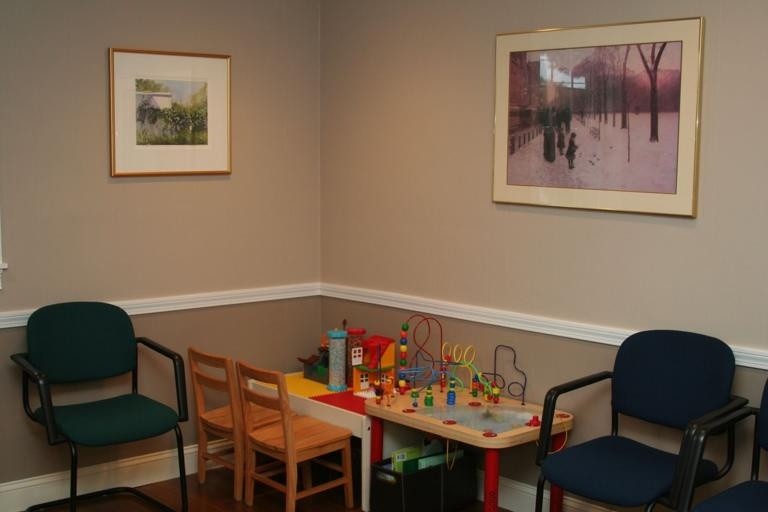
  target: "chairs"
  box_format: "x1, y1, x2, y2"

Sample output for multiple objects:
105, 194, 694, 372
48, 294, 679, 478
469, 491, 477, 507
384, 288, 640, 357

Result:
679, 375, 768, 511
10, 302, 192, 511
187, 344, 297, 500
537, 329, 749, 512
237, 361, 355, 511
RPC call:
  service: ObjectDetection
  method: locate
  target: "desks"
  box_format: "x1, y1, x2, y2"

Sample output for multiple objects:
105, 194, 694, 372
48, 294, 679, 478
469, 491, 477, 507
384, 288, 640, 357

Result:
248, 371, 442, 511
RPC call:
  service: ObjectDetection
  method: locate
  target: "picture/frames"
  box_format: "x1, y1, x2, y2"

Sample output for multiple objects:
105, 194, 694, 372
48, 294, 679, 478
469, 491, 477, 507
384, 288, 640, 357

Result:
108, 46, 235, 178
490, 17, 702, 218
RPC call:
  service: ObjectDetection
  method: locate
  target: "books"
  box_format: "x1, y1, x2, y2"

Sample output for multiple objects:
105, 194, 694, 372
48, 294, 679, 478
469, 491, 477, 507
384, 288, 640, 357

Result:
377, 439, 461, 482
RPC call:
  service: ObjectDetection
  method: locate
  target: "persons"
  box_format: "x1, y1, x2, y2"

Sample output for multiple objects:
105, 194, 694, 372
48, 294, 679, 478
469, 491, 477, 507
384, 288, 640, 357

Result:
539, 100, 578, 170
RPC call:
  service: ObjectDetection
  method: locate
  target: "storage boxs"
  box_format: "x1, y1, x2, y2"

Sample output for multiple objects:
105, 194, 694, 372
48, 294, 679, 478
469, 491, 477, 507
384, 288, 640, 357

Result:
368, 456, 477, 512
